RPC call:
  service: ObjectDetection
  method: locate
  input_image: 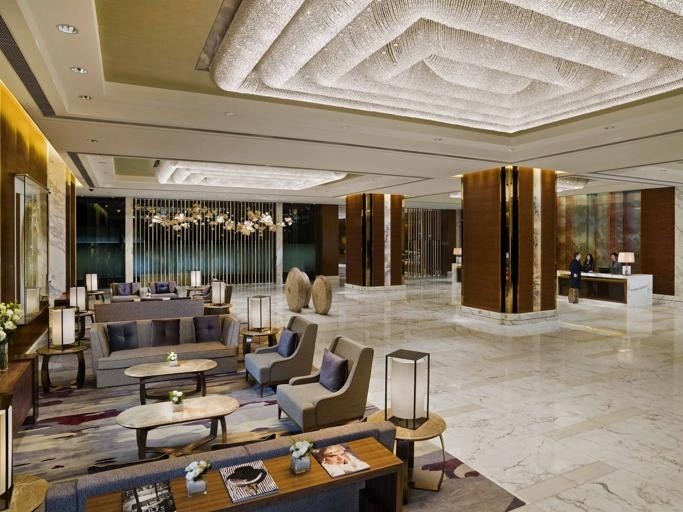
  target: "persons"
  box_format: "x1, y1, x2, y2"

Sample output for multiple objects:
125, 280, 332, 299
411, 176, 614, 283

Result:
313, 444, 368, 477
608, 253, 622, 275
581, 252, 595, 272
567, 252, 593, 304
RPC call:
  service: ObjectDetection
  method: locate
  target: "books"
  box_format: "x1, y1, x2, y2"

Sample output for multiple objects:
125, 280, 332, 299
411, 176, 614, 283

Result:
121, 480, 177, 511
309, 441, 371, 479
218, 458, 279, 504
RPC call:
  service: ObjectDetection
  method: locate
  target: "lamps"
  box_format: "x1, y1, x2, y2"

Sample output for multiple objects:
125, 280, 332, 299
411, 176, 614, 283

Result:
616, 248, 636, 275
452, 247, 462, 259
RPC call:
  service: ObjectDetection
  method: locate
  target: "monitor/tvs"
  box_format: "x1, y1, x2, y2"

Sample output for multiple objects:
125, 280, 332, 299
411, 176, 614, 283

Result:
599, 267, 610, 273
